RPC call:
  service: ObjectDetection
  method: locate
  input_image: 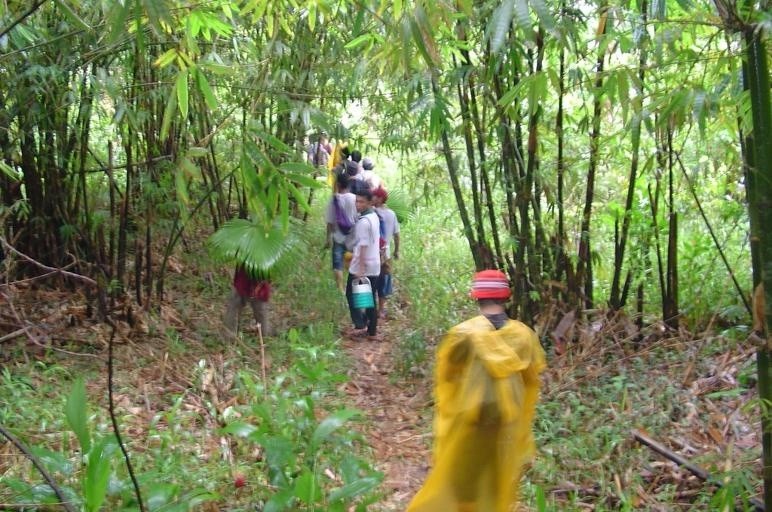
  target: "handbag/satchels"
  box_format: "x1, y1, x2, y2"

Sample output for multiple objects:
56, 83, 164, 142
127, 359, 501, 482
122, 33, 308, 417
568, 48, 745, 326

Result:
380, 236, 390, 264
335, 208, 354, 235
383, 257, 393, 274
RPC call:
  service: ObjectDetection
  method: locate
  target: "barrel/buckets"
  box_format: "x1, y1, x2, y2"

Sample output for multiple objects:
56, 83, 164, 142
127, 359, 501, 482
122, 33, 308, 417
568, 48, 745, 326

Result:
350, 276, 375, 310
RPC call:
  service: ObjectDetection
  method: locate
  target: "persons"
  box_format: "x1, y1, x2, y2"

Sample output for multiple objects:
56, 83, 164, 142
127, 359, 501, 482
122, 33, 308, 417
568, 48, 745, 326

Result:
223, 255, 271, 338
405, 268, 547, 510
306, 130, 404, 341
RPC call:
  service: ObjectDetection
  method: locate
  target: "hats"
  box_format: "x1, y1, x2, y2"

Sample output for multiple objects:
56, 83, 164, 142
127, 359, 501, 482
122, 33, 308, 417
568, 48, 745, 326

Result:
367, 185, 387, 200
340, 147, 351, 155
470, 269, 511, 299
